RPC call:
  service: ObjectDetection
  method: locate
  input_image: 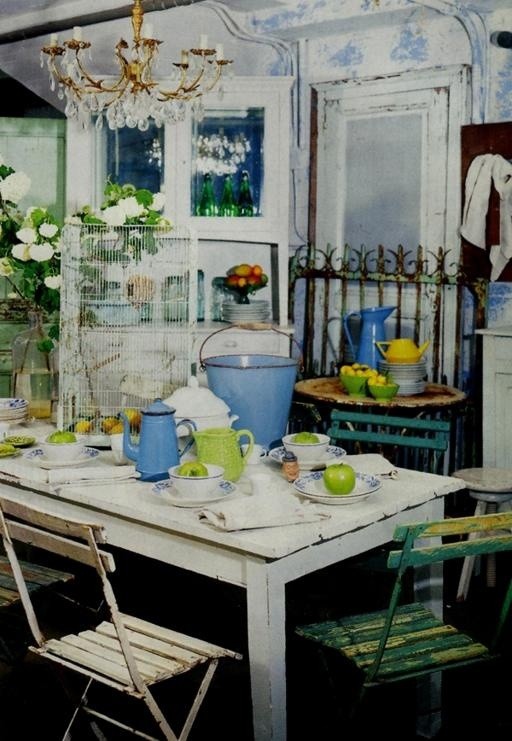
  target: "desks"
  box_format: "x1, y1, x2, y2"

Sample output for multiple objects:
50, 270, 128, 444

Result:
293, 376, 466, 461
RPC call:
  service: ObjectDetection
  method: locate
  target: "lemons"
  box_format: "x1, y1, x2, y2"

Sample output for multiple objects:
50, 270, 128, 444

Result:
340, 363, 397, 387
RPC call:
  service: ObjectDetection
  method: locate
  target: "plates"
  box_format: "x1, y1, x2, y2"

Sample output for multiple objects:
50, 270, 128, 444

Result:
25, 432, 100, 469
270, 445, 345, 465
378, 360, 428, 398
293, 468, 385, 507
222, 301, 270, 324
0, 397, 30, 426
150, 478, 241, 509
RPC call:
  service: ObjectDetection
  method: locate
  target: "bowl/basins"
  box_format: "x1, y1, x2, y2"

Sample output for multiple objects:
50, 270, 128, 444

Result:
282, 431, 331, 459
168, 461, 227, 498
337, 375, 367, 397
366, 382, 399, 399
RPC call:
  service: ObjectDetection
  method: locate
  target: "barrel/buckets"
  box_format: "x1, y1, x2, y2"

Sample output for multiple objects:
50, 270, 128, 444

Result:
198, 322, 304, 455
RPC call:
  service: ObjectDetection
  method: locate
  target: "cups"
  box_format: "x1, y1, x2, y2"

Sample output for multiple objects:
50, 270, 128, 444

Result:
241, 443, 266, 468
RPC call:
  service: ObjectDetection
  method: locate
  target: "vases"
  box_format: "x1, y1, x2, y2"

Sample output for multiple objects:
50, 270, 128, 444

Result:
5, 309, 59, 414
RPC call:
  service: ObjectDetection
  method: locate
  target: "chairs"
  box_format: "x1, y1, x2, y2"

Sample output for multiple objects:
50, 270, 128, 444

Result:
290, 503, 511, 735
319, 405, 453, 478
0, 494, 248, 741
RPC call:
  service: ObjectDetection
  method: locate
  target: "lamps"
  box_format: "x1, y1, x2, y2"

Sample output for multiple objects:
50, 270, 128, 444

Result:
38, 1, 238, 138
138, 126, 257, 180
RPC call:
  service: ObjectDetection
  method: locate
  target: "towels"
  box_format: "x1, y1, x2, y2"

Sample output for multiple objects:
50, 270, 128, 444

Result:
457, 149, 511, 285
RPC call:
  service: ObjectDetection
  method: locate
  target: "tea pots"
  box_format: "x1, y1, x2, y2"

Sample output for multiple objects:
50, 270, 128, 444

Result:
192, 428, 256, 485
375, 337, 429, 363
342, 305, 398, 372
118, 398, 200, 481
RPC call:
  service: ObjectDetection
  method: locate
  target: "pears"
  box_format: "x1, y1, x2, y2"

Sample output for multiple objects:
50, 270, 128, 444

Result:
76, 408, 141, 435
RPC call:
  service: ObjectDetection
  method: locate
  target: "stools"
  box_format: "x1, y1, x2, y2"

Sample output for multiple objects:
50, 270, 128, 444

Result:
443, 466, 512, 602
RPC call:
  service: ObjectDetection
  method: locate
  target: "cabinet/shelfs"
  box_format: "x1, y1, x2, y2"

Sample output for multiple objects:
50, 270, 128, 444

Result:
54, 65, 299, 424
473, 322, 510, 520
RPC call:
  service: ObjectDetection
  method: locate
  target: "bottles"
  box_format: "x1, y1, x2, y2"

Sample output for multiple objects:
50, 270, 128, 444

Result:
198, 270, 205, 321
214, 277, 230, 322
280, 453, 300, 482
12, 310, 58, 419
197, 171, 253, 216
166, 276, 184, 323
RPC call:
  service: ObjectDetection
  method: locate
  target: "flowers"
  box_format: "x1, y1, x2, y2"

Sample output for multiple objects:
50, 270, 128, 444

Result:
2, 157, 176, 403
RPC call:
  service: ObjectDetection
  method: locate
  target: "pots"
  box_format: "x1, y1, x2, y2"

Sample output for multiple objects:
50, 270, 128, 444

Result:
162, 377, 239, 439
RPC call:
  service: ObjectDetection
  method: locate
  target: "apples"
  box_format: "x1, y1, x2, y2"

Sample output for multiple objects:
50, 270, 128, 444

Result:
49, 431, 76, 443
179, 461, 208, 477
227, 264, 263, 287
323, 462, 356, 495
292, 432, 319, 443
